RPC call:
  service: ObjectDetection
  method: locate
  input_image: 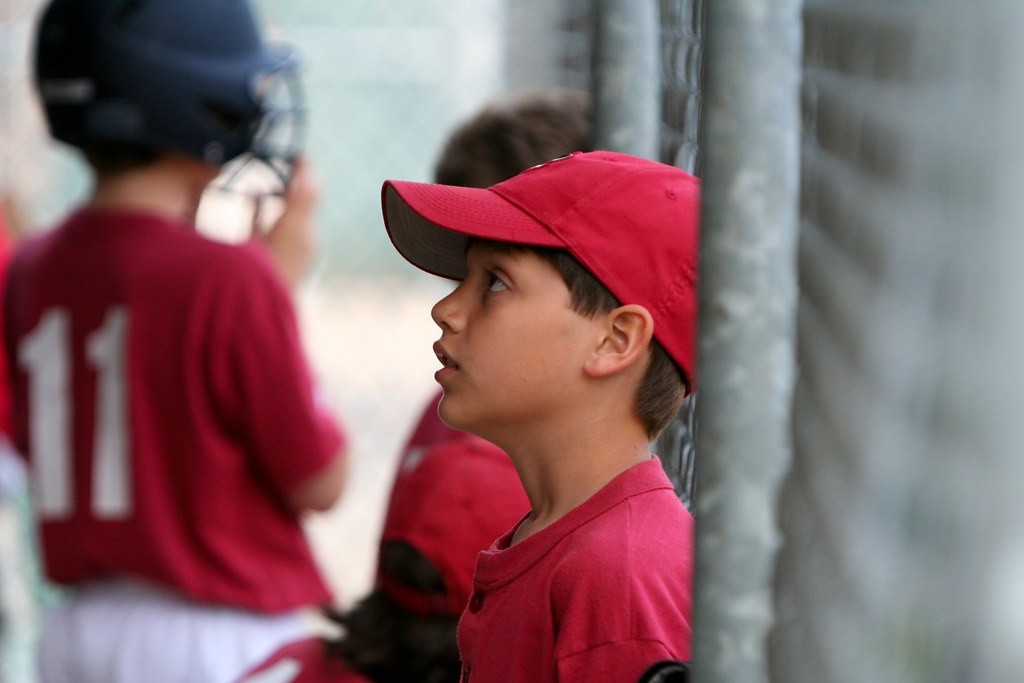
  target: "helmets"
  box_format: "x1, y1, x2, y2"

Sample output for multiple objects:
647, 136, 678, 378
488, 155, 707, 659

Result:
36, 0, 265, 161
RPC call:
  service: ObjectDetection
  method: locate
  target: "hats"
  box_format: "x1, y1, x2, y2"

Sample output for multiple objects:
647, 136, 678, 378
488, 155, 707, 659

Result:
380, 150, 702, 397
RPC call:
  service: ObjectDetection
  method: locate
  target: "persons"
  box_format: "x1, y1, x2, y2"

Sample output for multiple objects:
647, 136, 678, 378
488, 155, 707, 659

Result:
372, 103, 591, 614
0, 1, 353, 683
381, 148, 702, 683
